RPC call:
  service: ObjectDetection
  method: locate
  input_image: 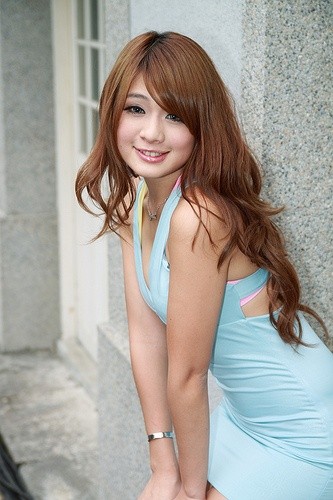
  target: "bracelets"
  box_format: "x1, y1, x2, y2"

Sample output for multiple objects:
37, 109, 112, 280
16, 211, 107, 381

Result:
145, 432, 174, 442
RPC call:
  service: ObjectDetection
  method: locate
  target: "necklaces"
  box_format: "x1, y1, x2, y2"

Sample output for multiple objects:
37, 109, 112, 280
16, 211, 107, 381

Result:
145, 189, 170, 222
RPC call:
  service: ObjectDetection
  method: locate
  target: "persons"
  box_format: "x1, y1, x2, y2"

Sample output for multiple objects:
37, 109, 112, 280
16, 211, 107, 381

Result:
73, 31, 333, 500
